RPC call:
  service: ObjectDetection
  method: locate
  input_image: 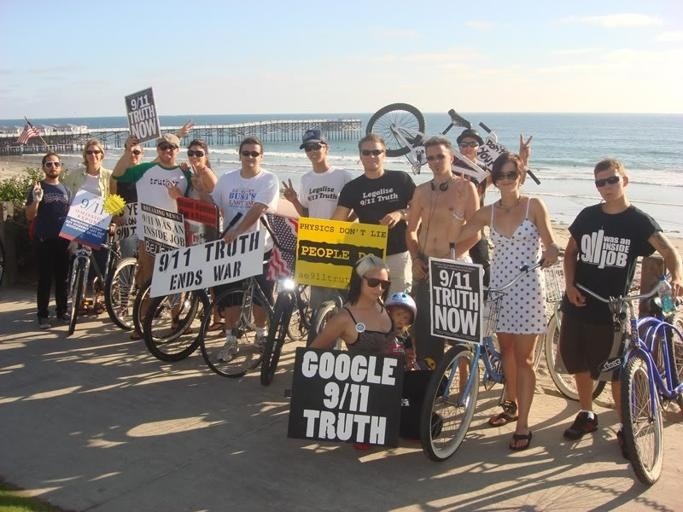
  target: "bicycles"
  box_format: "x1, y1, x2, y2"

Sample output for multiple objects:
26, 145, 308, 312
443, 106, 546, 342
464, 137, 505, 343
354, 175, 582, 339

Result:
362, 101, 541, 194
107, 215, 314, 379
530, 249, 609, 405
570, 267, 682, 488
63, 204, 136, 336
421, 245, 561, 462
259, 229, 345, 388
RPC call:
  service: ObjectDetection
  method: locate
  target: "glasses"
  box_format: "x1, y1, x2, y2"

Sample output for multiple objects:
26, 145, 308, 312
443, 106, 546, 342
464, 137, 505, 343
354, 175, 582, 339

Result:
460, 141, 477, 147
361, 150, 383, 155
241, 151, 261, 157
86, 150, 100, 154
187, 150, 203, 157
45, 161, 60, 168
305, 143, 321, 151
133, 150, 140, 155
362, 275, 390, 288
494, 171, 517, 180
159, 144, 175, 149
427, 155, 444, 160
594, 176, 618, 187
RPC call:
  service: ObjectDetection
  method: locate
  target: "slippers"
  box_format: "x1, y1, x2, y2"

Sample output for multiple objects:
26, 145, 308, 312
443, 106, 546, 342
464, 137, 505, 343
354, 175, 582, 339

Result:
509, 431, 531, 450
488, 413, 518, 427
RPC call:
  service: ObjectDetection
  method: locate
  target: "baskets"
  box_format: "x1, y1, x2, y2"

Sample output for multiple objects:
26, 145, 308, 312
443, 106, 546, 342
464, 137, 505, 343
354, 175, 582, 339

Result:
483, 291, 505, 338
541, 265, 566, 303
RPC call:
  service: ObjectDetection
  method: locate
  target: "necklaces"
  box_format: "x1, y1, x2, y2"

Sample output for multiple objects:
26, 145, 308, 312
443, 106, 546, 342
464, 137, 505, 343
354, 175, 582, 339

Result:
496, 195, 521, 214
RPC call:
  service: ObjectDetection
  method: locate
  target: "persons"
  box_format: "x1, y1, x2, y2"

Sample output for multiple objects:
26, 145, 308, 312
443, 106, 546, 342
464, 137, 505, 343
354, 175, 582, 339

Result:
25, 153, 71, 329
309, 253, 416, 368
406, 137, 482, 407
165, 138, 223, 331
63, 140, 113, 315
448, 153, 559, 450
329, 134, 419, 297
188, 136, 279, 363
109, 132, 193, 339
283, 129, 354, 314
116, 121, 193, 315
383, 290, 417, 370
450, 129, 533, 304
559, 160, 683, 459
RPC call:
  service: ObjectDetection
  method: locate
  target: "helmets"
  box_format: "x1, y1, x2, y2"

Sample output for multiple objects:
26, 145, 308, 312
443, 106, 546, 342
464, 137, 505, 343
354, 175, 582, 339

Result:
383, 292, 416, 323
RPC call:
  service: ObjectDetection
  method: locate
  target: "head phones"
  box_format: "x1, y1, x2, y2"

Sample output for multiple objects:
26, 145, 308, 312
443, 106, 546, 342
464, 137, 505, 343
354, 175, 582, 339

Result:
430, 174, 455, 191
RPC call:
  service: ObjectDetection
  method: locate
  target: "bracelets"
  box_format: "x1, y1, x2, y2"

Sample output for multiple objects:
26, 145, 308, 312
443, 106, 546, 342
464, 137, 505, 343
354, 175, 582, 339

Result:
411, 254, 419, 260
400, 209, 407, 217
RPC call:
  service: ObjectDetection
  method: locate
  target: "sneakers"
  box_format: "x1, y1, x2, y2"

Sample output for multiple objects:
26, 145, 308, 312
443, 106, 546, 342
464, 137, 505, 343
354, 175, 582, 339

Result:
254, 334, 277, 353
616, 427, 630, 460
38, 317, 51, 329
216, 341, 239, 362
564, 412, 597, 439
58, 312, 71, 323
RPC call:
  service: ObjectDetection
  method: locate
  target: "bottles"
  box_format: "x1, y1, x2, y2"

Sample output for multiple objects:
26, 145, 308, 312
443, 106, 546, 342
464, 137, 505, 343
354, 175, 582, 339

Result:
657, 274, 674, 313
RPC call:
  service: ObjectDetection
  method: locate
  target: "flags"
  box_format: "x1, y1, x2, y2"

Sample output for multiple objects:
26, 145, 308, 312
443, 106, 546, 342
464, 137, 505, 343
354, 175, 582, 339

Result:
14, 122, 40, 145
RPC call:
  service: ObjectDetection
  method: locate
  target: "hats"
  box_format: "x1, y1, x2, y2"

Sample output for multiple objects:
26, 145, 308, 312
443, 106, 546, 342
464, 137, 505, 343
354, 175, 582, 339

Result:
157, 133, 180, 148
456, 129, 483, 146
299, 129, 327, 149
